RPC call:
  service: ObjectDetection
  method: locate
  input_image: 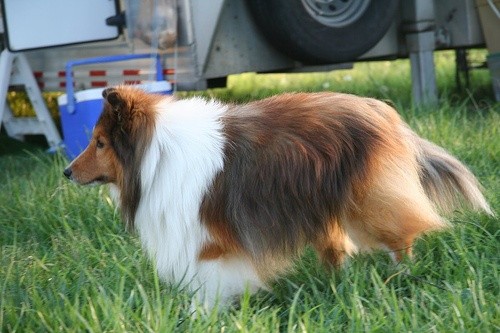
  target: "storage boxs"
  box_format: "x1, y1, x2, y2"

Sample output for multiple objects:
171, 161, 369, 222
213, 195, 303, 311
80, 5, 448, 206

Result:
476, 1, 500, 102
60, 55, 170, 162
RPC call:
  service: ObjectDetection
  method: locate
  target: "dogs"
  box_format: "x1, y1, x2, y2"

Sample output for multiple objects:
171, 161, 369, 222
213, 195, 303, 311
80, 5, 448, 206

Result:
62, 84, 495, 322
